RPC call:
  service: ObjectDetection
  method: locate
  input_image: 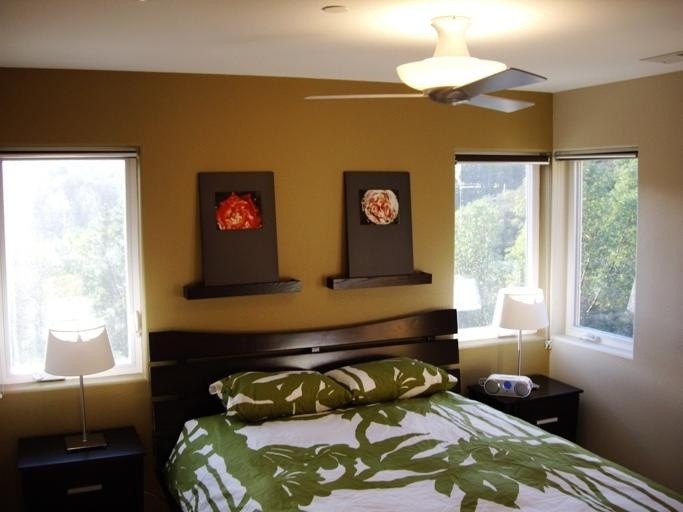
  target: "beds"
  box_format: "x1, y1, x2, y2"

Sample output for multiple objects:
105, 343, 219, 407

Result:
148, 309, 683, 512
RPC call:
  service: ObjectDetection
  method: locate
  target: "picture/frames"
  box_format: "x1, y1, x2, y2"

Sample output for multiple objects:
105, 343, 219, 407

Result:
198, 171, 279, 287
343, 170, 414, 278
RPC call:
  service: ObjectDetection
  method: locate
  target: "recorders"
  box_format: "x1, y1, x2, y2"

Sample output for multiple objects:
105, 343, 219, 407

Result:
484, 374, 533, 399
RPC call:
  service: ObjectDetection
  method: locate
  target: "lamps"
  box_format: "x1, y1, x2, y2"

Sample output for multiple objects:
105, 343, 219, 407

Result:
42, 323, 115, 452
492, 285, 551, 389
396, 16, 507, 92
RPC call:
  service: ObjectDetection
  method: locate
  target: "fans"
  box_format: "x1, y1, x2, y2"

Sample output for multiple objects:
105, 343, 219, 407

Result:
301, 12, 548, 114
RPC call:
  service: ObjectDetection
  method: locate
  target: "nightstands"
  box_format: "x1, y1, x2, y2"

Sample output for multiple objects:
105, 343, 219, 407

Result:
16, 424, 147, 512
467, 373, 584, 445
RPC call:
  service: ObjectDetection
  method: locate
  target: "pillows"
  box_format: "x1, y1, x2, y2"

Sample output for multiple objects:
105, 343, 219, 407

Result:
209, 368, 352, 423
323, 357, 459, 406
